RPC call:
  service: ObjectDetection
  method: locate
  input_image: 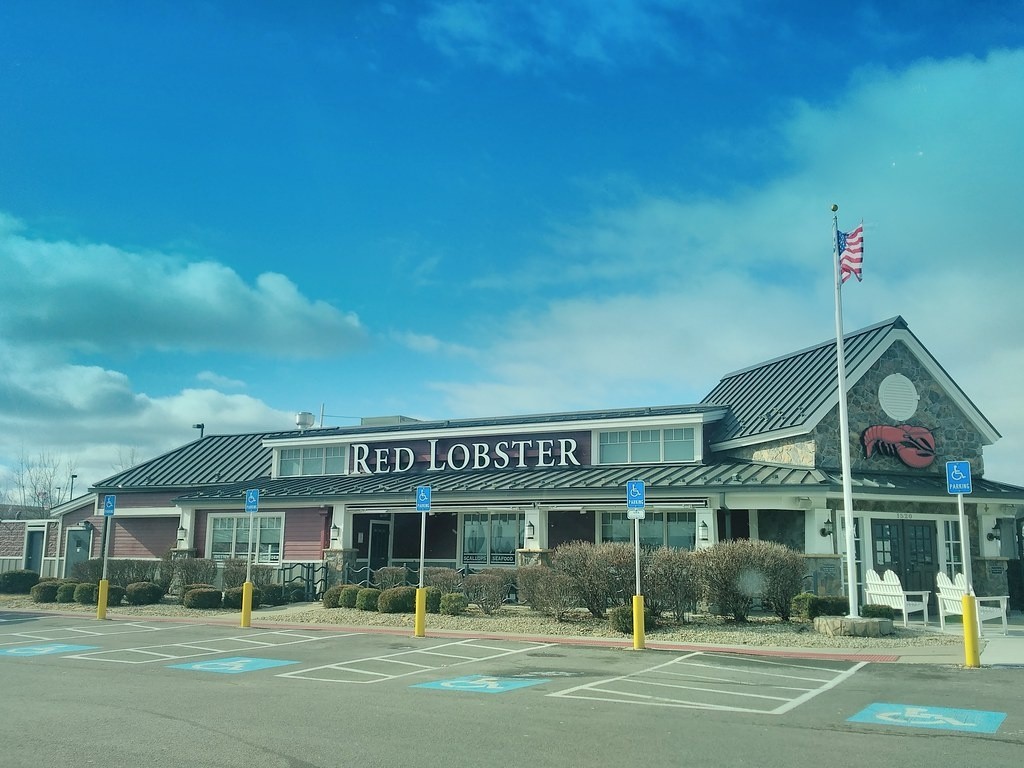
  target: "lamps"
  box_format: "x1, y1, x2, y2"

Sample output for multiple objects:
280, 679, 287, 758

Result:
330, 523, 340, 541
986, 523, 1001, 542
819, 519, 833, 538
177, 524, 187, 541
525, 521, 534, 540
698, 519, 708, 541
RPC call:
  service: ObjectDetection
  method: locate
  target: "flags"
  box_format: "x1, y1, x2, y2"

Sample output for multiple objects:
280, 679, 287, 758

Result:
834, 221, 864, 284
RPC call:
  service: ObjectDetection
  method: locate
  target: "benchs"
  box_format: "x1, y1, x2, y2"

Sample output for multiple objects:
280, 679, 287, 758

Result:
863, 569, 931, 628
934, 572, 1010, 636
